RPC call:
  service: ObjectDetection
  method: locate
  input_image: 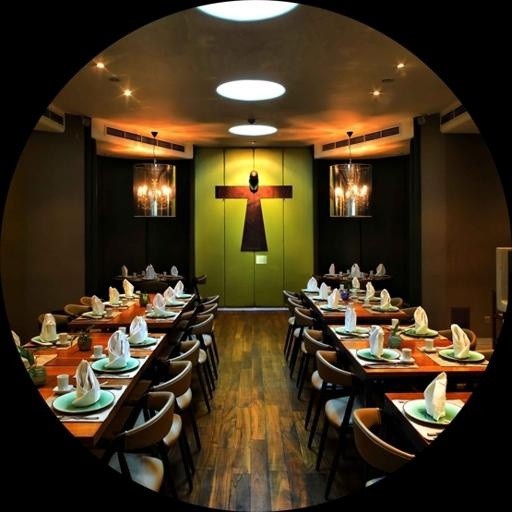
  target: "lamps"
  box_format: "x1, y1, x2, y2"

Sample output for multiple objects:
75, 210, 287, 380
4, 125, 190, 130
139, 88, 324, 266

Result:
330, 132, 373, 217
133, 132, 176, 217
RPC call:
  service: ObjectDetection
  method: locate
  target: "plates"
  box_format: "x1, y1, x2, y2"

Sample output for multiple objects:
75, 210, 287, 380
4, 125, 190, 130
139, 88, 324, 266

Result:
17, 283, 193, 423
300, 274, 488, 364
116, 262, 183, 283
402, 397, 462, 426
325, 260, 391, 283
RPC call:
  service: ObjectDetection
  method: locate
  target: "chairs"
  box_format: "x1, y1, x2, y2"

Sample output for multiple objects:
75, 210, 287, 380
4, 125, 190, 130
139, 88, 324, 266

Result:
11, 263, 219, 499
280, 261, 493, 503
